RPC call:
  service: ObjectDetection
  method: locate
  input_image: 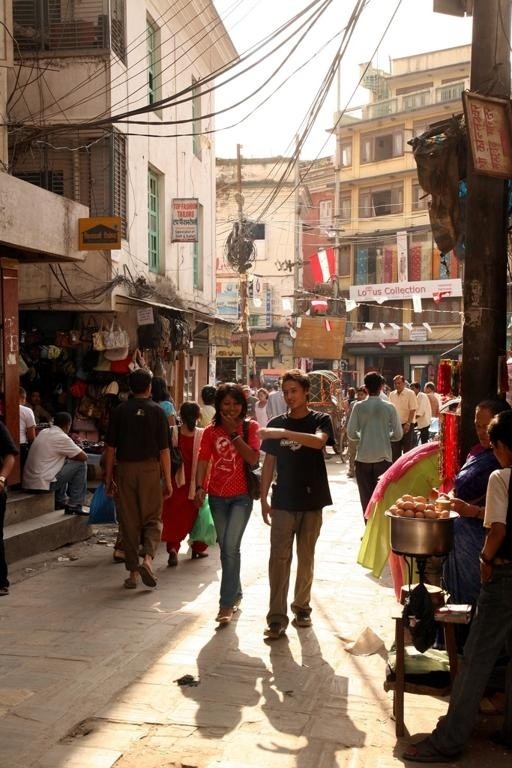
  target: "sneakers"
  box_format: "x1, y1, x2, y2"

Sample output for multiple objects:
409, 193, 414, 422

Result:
124, 578, 137, 591
139, 562, 159, 588
168, 553, 177, 566
192, 549, 209, 559
114, 549, 126, 561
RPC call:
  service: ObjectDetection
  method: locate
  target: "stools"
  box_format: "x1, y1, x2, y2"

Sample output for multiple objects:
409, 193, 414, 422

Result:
383, 604, 473, 738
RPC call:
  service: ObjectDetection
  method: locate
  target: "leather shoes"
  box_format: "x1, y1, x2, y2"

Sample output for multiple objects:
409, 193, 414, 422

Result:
264, 622, 287, 639
232, 605, 238, 612
215, 609, 233, 623
295, 611, 311, 626
55, 499, 66, 509
65, 504, 90, 516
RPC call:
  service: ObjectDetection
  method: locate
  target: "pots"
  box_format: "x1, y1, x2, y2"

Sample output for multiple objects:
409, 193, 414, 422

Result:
383, 506, 460, 558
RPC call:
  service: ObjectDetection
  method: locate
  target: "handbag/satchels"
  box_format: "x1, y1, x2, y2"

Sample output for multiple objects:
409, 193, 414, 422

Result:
245, 460, 264, 501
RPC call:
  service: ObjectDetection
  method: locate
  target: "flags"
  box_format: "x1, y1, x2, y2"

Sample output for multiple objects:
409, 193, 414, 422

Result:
304, 246, 339, 291
432, 289, 452, 306
308, 298, 331, 312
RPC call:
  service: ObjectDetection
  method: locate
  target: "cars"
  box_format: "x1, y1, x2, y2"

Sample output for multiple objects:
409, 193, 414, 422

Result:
428, 398, 462, 435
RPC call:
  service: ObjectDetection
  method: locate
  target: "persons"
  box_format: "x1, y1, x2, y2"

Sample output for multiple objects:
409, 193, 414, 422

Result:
103, 368, 175, 590
402, 407, 511, 764
193, 382, 263, 621
99, 369, 446, 568
22, 410, 93, 517
26, 388, 52, 429
18, 384, 38, 460
433, 396, 511, 716
0, 421, 19, 598
254, 372, 337, 638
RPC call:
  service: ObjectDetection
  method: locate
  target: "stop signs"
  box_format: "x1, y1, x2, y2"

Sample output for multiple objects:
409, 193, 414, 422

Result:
310, 297, 328, 312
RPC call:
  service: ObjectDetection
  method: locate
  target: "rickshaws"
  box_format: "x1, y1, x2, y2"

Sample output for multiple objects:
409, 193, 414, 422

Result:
306, 368, 352, 464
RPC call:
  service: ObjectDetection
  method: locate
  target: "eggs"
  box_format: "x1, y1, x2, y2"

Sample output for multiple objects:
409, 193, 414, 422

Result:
389, 494, 448, 518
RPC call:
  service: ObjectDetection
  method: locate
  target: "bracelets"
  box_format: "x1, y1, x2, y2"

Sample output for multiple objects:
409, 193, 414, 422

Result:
478, 553, 495, 570
0, 475, 9, 484
476, 505, 483, 522
460, 504, 470, 518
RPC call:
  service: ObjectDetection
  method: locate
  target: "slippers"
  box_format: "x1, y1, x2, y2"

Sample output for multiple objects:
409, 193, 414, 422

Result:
403, 736, 460, 762
